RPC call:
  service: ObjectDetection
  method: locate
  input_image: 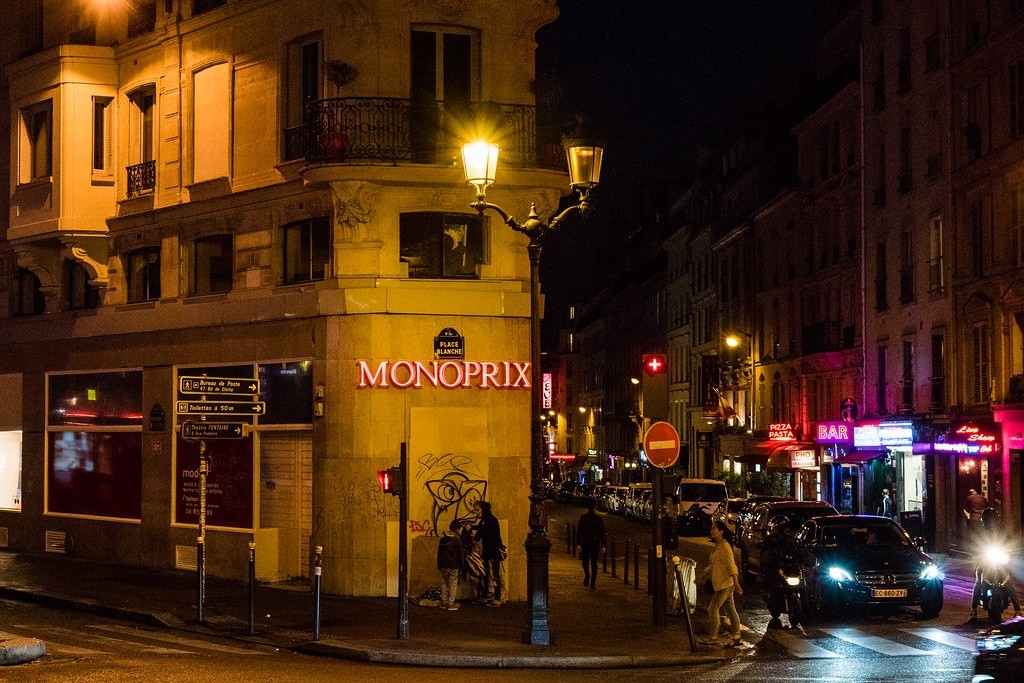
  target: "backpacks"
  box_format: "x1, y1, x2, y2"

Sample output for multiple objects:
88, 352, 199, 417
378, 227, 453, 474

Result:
442, 531, 460, 561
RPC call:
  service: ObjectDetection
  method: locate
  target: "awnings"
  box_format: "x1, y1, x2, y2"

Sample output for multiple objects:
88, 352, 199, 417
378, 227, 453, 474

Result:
834, 448, 892, 465
765, 442, 821, 470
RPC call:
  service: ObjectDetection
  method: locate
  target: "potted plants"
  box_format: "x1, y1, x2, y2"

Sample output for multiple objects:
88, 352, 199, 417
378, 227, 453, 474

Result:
320, 60, 358, 162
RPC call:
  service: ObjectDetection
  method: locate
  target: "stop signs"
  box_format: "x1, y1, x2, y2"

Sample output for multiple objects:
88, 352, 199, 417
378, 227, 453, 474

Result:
643, 420, 680, 468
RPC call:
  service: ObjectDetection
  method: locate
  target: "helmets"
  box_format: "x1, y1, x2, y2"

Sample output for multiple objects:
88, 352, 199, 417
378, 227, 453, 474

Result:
981, 507, 1001, 527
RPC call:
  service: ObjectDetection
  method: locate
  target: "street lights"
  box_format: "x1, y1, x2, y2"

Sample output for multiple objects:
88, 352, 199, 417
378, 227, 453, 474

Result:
459, 133, 605, 645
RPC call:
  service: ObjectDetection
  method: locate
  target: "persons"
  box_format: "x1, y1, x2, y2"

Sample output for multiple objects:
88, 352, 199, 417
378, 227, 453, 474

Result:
879, 488, 895, 518
436, 519, 464, 610
575, 499, 607, 591
466, 501, 505, 607
661, 492, 684, 551
963, 488, 988, 525
760, 515, 816, 624
694, 521, 744, 647
864, 531, 879, 545
969, 527, 1021, 620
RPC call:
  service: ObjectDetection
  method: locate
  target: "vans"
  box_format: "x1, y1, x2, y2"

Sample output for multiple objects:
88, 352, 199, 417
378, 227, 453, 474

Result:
675, 477, 728, 519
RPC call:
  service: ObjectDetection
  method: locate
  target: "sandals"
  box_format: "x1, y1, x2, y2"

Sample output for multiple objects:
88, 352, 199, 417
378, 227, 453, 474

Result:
702, 638, 720, 646
724, 637, 743, 648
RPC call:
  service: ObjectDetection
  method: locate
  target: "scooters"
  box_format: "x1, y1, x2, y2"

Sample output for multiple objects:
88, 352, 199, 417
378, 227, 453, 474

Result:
973, 557, 1013, 626
758, 546, 812, 628
682, 496, 712, 539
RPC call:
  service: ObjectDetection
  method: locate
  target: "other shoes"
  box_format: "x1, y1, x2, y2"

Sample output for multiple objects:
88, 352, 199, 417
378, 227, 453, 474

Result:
591, 583, 596, 590
1013, 611, 1022, 618
583, 574, 590, 586
486, 600, 501, 606
448, 603, 459, 611
439, 603, 448, 609
969, 612, 978, 620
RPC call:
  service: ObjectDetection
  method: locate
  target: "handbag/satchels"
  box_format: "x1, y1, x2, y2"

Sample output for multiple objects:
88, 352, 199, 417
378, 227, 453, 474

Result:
704, 578, 715, 596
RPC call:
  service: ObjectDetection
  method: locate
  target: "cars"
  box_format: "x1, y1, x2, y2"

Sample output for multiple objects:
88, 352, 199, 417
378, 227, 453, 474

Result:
974, 614, 1023, 683
542, 478, 677, 524
794, 514, 944, 624
710, 494, 840, 577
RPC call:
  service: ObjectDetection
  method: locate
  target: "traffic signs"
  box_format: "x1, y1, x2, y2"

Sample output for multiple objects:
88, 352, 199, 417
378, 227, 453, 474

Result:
181, 421, 245, 440
176, 400, 266, 415
176, 375, 260, 397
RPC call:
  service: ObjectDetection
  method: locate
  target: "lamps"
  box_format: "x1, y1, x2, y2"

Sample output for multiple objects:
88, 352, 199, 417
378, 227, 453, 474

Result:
148, 404, 166, 432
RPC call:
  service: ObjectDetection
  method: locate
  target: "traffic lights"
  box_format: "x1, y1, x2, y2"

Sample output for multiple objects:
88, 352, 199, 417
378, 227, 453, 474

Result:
642, 353, 671, 418
377, 469, 402, 494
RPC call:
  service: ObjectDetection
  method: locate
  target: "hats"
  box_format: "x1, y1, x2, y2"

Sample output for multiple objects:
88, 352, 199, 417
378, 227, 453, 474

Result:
450, 520, 463, 530
